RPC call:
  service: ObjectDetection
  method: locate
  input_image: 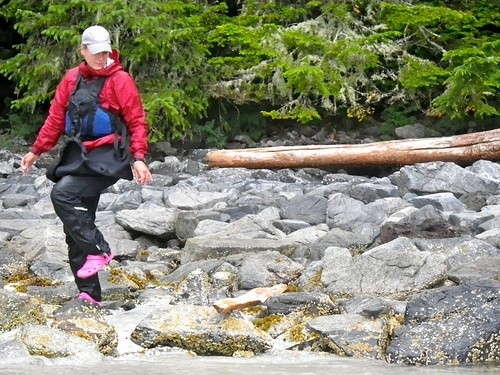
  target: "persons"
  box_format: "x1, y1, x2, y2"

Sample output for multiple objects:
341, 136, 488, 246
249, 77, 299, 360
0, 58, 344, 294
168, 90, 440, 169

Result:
20, 25, 153, 306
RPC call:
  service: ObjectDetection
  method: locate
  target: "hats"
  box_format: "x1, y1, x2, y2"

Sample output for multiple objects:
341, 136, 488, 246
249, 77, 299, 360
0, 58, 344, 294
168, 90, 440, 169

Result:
81, 24, 113, 55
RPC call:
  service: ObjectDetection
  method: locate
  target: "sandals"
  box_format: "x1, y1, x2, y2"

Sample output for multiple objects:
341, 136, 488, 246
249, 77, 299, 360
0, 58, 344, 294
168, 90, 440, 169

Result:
77, 292, 101, 306
78, 251, 114, 279
